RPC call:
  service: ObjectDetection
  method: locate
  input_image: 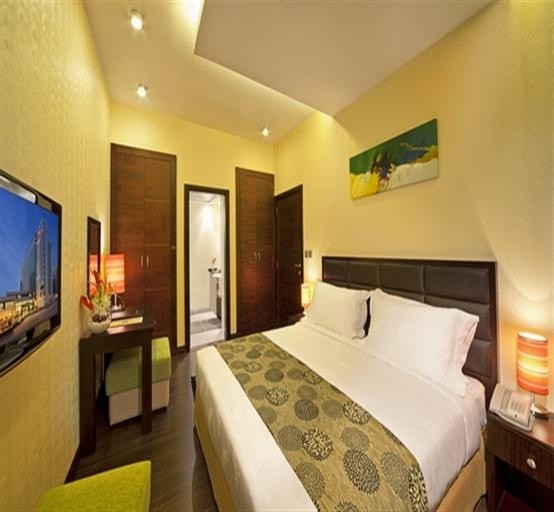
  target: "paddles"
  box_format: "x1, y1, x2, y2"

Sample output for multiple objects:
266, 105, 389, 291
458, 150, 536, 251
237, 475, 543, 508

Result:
35, 459, 152, 512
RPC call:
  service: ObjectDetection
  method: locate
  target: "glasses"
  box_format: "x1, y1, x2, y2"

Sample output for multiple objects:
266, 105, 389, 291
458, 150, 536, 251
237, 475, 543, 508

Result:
104, 253, 126, 311
301, 282, 314, 315
517, 332, 550, 420
88, 254, 98, 298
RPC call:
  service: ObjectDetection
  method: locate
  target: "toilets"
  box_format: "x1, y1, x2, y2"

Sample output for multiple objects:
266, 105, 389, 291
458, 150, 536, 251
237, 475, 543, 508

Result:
489, 383, 534, 432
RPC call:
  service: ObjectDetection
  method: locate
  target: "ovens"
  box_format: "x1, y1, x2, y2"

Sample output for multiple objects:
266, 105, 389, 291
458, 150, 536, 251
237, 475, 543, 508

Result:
192, 253, 500, 512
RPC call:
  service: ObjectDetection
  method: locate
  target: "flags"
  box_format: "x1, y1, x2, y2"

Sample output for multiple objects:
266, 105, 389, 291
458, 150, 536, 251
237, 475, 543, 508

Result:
87, 320, 112, 333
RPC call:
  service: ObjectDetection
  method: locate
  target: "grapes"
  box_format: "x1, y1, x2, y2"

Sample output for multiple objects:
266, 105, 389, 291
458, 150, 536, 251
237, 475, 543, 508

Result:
486, 411, 554, 512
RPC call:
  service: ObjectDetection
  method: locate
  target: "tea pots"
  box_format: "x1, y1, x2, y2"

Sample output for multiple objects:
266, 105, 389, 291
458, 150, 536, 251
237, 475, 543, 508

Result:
86, 216, 102, 300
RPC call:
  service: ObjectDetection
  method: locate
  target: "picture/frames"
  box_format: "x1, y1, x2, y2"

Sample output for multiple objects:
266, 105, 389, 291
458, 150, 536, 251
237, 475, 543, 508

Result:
78, 320, 155, 456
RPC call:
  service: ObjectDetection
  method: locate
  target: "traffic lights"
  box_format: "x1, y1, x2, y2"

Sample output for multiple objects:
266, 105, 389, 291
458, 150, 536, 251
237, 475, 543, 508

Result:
307, 278, 369, 340
359, 288, 480, 396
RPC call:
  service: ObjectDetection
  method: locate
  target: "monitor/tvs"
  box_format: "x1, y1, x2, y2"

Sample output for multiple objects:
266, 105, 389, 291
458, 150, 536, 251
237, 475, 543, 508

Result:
108, 309, 144, 327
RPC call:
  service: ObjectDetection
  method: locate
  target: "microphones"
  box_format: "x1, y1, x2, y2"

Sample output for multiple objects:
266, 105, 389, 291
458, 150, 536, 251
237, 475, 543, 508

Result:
105, 337, 171, 427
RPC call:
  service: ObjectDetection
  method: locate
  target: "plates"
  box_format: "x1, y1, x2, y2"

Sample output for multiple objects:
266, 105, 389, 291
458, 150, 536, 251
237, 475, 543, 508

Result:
235, 166, 275, 336
111, 142, 177, 354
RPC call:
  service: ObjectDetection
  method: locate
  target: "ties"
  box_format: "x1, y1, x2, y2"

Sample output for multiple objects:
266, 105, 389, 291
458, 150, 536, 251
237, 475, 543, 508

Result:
0, 167, 62, 378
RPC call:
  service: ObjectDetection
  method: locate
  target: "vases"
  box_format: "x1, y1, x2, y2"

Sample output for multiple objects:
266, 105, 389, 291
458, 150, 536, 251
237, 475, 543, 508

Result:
80, 269, 113, 320
